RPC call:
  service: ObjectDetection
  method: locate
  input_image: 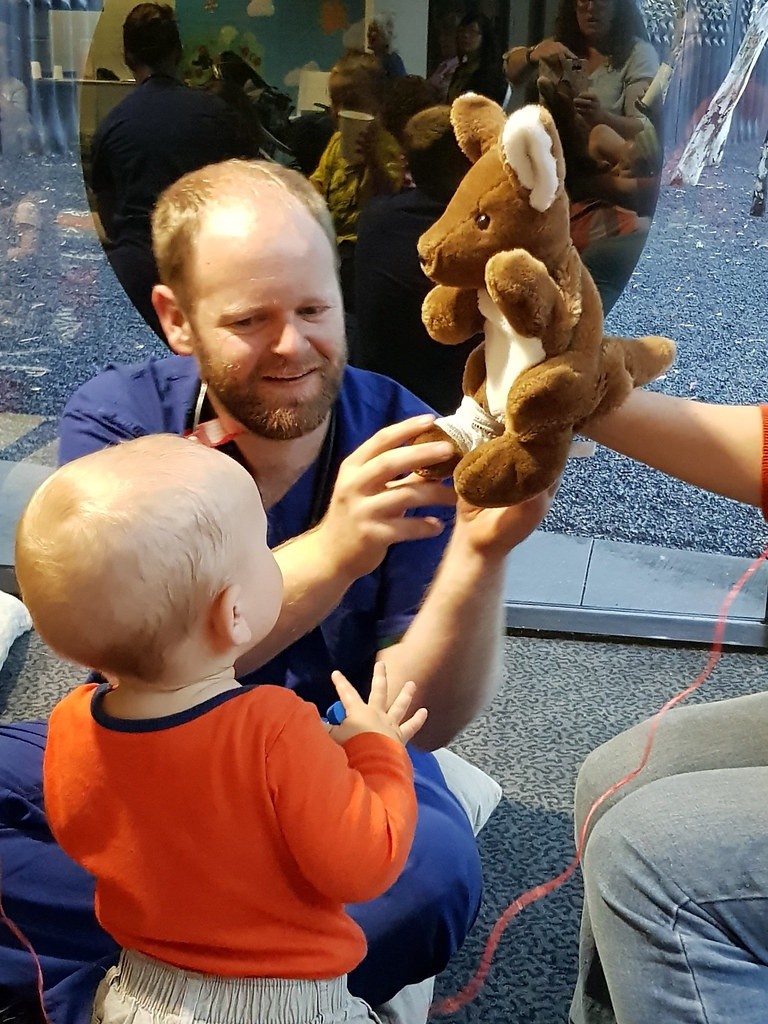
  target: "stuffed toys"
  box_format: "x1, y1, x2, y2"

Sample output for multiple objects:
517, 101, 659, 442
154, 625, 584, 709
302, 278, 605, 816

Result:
414, 92, 677, 508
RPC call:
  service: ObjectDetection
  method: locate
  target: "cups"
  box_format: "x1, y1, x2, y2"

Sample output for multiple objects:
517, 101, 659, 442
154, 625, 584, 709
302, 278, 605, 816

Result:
53, 65, 63, 80
31, 62, 42, 80
338, 111, 373, 159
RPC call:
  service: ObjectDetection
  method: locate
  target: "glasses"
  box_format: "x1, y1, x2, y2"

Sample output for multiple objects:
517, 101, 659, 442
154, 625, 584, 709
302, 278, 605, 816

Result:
456, 26, 483, 38
578, 0, 609, 8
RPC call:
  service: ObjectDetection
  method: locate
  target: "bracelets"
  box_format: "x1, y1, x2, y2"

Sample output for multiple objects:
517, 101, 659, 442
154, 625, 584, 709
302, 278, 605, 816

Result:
525, 44, 538, 65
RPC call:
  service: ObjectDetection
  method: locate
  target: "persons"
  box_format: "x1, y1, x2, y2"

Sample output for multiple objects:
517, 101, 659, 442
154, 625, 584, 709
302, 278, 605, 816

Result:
0, 157, 562, 1024
568, 389, 768, 1024
17, 434, 428, 1024
80, 0, 663, 416
